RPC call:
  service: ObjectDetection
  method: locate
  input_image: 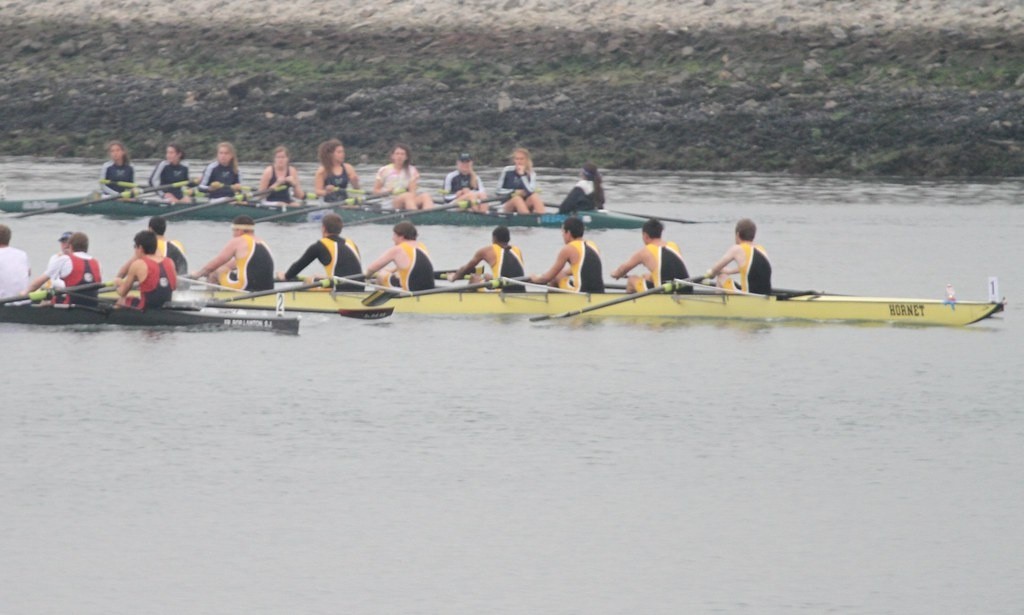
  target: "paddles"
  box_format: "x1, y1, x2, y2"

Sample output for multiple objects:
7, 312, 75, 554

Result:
159, 185, 288, 218
211, 181, 259, 191
202, 300, 395, 320
99, 179, 148, 189
718, 271, 742, 290
9, 178, 200, 219
622, 275, 716, 287
433, 273, 484, 281
219, 274, 367, 302
290, 191, 318, 199
361, 275, 541, 307
529, 271, 711, 322
272, 276, 322, 283
253, 188, 408, 223
333, 186, 372, 195
546, 279, 626, 290
367, 264, 485, 279
98, 273, 207, 294
543, 202, 699, 224
349, 188, 540, 227
183, 189, 208, 197
0, 280, 116, 304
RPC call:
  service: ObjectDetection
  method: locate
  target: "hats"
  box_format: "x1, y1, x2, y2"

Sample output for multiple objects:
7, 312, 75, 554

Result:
58, 232, 72, 240
458, 152, 471, 161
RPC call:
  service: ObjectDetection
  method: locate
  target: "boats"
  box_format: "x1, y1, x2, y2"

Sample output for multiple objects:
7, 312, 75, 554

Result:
0, 290, 301, 337
0, 189, 650, 231
90, 271, 1007, 331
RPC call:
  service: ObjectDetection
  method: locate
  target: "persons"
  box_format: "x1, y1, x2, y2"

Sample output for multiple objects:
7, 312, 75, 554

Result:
529, 216, 606, 295
366, 218, 435, 292
118, 215, 187, 276
554, 162, 605, 215
703, 216, 773, 297
0, 223, 31, 302
314, 139, 366, 209
443, 149, 490, 214
448, 226, 528, 293
97, 138, 139, 203
257, 144, 306, 208
21, 233, 102, 307
113, 230, 177, 310
188, 215, 274, 292
615, 220, 695, 295
276, 212, 364, 292
41, 232, 73, 289
199, 142, 254, 207
149, 143, 204, 204
372, 141, 433, 214
495, 147, 545, 214
